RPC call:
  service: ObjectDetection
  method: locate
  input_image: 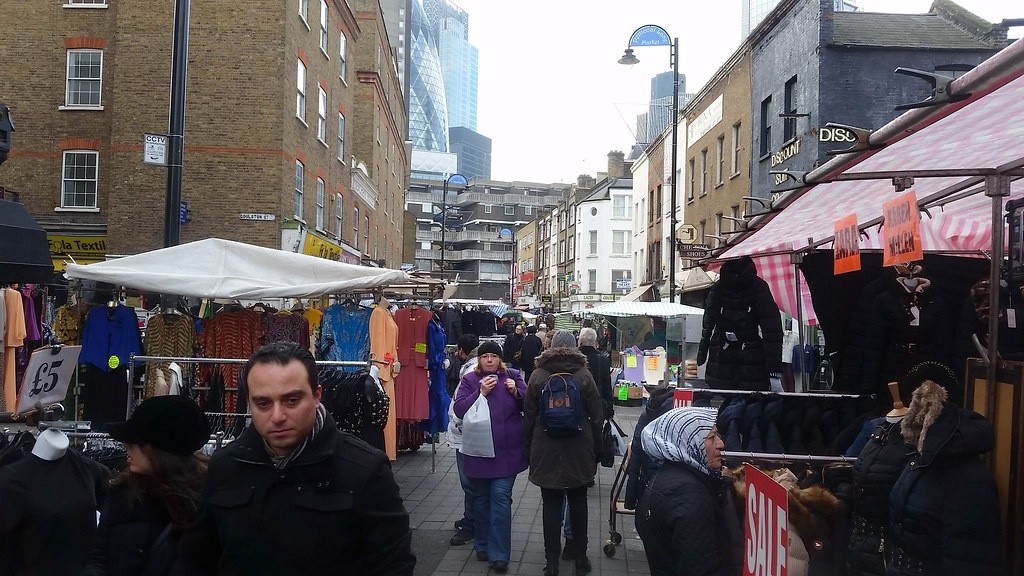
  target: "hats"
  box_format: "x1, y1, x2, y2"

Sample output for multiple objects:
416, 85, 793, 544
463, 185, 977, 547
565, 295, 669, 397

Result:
549, 329, 578, 350
538, 324, 550, 329
478, 341, 503, 357
102, 395, 213, 457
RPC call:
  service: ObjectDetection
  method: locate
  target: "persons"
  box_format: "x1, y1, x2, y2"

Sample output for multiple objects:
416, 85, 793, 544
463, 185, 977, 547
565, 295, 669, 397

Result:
640, 332, 659, 351
637, 407, 744, 576
524, 331, 603, 576
195, 340, 416, 576
577, 328, 614, 487
453, 341, 527, 571
82, 395, 211, 576
496, 319, 579, 379
450, 334, 479, 545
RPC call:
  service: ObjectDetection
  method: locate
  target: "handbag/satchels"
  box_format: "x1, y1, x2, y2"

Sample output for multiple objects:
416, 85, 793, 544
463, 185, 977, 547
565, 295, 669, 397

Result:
601, 416, 626, 468
459, 394, 497, 460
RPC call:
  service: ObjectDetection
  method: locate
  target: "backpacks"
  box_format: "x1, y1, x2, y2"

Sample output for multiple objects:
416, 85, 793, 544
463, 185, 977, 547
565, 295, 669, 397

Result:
536, 371, 589, 440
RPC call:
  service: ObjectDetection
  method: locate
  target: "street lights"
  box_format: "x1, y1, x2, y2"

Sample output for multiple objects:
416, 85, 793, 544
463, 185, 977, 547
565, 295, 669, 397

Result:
497, 229, 515, 308
440, 174, 472, 282
617, 24, 679, 302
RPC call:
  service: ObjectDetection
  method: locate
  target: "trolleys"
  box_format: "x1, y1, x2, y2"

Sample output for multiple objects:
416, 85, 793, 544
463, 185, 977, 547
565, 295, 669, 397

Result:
600, 437, 643, 557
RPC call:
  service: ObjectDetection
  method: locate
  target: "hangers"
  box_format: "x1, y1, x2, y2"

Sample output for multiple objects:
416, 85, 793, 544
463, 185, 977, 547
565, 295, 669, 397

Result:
777, 453, 786, 476
163, 298, 190, 316
208, 300, 211, 309
841, 456, 847, 462
200, 411, 248, 456
317, 360, 362, 380
722, 451, 731, 471
464, 301, 481, 312
448, 303, 456, 310
438, 305, 444, 310
806, 454, 814, 477
341, 292, 368, 309
750, 451, 760, 468
146, 304, 161, 318
4, 414, 36, 448
214, 303, 243, 313
107, 288, 119, 308
64, 432, 127, 456
38, 403, 92, 430
247, 298, 268, 312
156, 356, 176, 370
410, 298, 418, 310
273, 297, 304, 316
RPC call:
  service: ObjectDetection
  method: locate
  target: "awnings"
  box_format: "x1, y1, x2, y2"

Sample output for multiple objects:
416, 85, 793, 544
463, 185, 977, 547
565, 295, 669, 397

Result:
619, 284, 660, 302
659, 266, 713, 298
0, 199, 53, 284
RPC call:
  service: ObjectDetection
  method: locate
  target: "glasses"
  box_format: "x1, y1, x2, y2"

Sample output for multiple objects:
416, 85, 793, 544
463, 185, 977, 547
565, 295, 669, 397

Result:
478, 354, 501, 360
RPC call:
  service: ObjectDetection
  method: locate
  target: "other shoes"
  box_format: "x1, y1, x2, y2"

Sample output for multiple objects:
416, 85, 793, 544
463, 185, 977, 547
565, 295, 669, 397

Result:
561, 538, 574, 561
541, 562, 560, 576
476, 551, 490, 561
451, 528, 476, 547
454, 518, 472, 530
491, 560, 509, 573
576, 557, 592, 576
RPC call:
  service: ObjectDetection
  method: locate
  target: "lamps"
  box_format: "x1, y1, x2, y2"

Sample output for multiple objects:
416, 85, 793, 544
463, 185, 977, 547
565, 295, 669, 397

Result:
333, 238, 342, 246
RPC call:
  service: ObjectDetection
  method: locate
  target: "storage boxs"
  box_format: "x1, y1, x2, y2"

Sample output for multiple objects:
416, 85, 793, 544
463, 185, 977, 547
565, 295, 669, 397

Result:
686, 359, 697, 370
614, 386, 644, 400
623, 355, 644, 385
645, 356, 667, 386
687, 369, 698, 379
613, 397, 643, 408
